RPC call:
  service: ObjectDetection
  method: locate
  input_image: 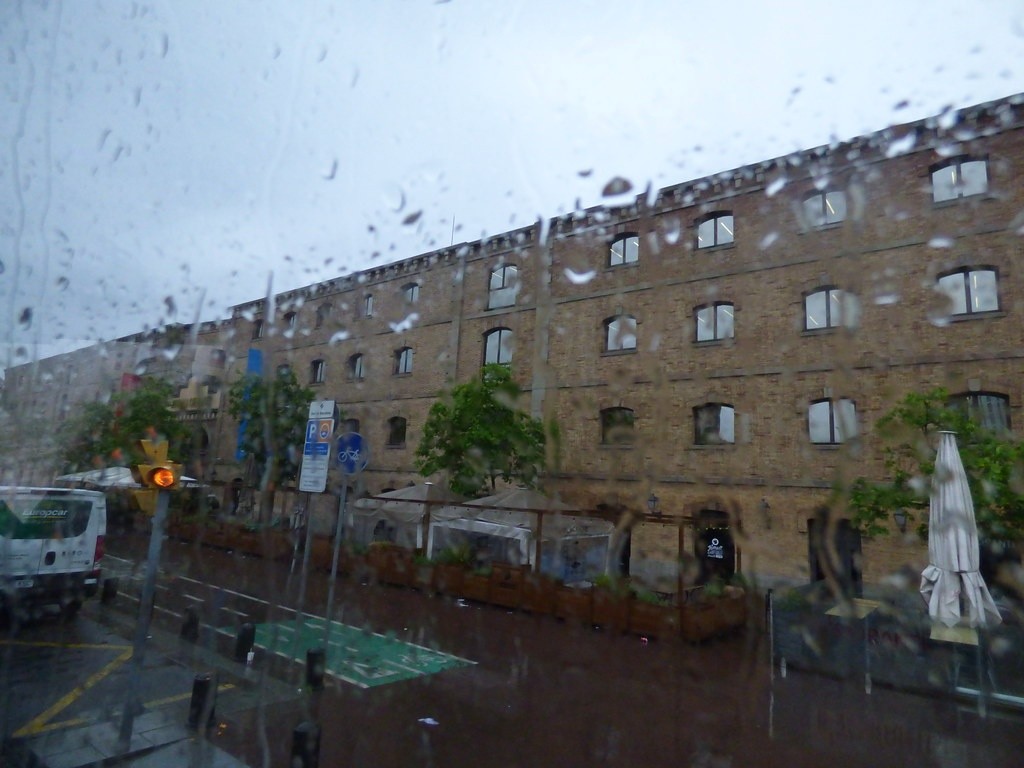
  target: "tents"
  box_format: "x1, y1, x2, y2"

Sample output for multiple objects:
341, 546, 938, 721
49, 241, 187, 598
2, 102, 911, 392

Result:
352, 482, 472, 551
427, 483, 615, 592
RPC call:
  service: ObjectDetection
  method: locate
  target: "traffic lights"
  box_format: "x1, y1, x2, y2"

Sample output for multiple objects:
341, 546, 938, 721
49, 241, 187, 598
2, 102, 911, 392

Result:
136, 462, 181, 492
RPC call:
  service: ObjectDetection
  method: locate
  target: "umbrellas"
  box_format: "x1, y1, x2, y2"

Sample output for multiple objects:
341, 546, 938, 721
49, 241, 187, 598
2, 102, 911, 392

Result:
56, 466, 208, 490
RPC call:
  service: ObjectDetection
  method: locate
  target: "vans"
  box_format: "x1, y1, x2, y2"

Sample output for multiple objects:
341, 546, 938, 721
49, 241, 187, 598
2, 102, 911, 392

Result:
0, 484, 109, 616
